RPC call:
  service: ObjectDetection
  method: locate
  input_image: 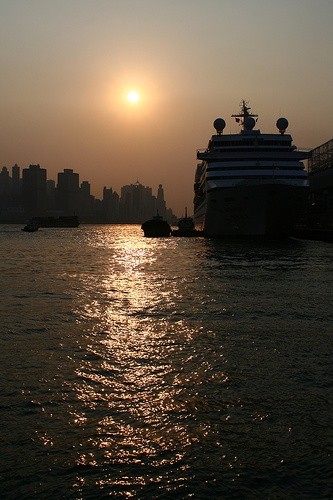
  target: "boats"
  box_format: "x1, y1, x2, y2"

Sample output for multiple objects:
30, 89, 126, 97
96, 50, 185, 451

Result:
141, 205, 173, 237
171, 205, 203, 238
27, 216, 80, 228
22, 224, 40, 232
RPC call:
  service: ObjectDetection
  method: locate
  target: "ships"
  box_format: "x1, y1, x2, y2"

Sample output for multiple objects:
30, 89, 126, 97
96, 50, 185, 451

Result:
192, 98, 311, 244
